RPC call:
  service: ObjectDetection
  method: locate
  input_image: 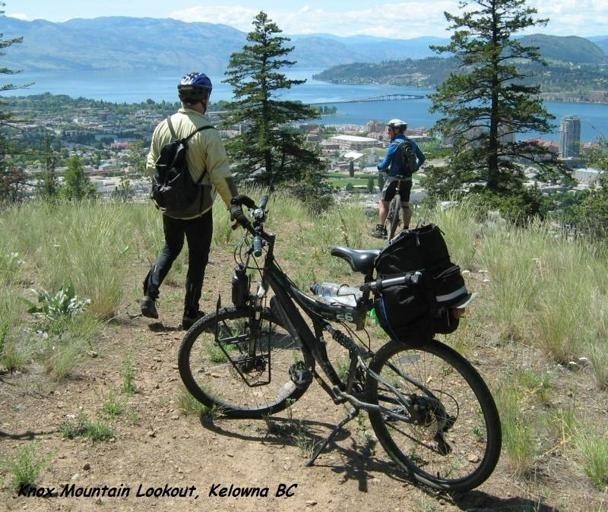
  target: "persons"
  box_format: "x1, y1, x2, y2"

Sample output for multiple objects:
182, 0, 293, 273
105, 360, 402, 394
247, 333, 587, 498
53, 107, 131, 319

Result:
371, 118, 426, 236
141, 72, 243, 330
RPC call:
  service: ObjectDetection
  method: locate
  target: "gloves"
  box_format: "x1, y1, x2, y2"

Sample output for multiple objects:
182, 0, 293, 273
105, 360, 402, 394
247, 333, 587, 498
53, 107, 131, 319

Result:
229, 206, 245, 231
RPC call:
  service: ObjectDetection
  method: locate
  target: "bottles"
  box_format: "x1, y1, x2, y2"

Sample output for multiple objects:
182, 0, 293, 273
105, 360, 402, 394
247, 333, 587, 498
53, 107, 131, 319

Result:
231, 264, 249, 306
310, 281, 362, 304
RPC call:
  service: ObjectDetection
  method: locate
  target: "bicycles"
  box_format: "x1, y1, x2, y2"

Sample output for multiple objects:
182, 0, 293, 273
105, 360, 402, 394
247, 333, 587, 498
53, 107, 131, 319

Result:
177, 181, 504, 493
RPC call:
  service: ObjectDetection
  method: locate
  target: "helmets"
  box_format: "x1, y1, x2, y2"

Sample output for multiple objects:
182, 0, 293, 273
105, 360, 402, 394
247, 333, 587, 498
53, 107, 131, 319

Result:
176, 70, 214, 96
387, 117, 409, 131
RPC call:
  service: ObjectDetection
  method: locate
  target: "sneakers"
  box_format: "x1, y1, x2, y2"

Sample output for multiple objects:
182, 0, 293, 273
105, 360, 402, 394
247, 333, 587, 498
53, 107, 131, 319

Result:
370, 228, 388, 239
181, 310, 207, 331
140, 294, 160, 320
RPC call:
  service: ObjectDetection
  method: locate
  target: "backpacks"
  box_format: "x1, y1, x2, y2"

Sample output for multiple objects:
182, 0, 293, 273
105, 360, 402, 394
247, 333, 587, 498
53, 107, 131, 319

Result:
390, 136, 420, 175
149, 114, 218, 214
373, 218, 474, 344
